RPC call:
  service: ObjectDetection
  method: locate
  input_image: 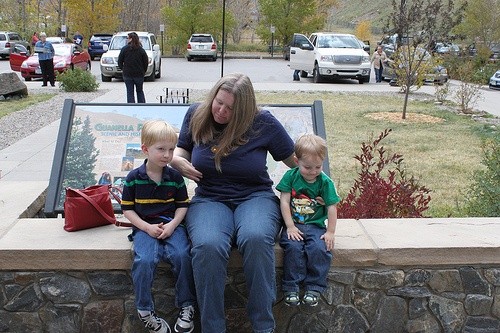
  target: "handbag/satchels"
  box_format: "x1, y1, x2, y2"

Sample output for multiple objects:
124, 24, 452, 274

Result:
63, 184, 133, 232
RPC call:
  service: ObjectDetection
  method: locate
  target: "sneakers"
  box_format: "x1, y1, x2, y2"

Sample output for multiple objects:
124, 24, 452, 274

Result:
174, 305, 198, 333
284, 291, 301, 306
138, 310, 172, 333
301, 290, 320, 307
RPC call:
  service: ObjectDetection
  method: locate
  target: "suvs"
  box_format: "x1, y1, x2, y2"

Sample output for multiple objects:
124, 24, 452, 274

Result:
289, 33, 372, 84
100, 31, 161, 81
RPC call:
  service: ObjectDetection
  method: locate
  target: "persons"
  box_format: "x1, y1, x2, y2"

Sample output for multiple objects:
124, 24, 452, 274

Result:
35, 32, 55, 87
294, 70, 300, 81
118, 32, 148, 103
275, 134, 342, 307
371, 46, 387, 83
121, 119, 198, 333
32, 32, 39, 53
73, 31, 83, 47
169, 73, 298, 333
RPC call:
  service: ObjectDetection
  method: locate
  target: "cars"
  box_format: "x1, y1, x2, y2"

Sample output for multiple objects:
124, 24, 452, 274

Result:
380, 45, 448, 85
187, 34, 218, 61
88, 33, 114, 60
488, 69, 500, 89
9, 43, 91, 81
375, 36, 500, 59
46, 37, 73, 43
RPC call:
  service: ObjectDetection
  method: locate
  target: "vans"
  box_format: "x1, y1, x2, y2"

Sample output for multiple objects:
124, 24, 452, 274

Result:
0, 31, 25, 58
